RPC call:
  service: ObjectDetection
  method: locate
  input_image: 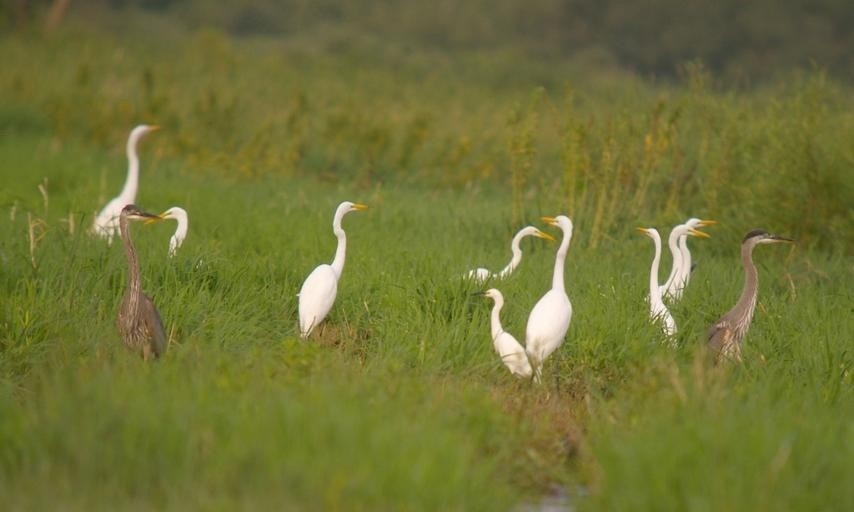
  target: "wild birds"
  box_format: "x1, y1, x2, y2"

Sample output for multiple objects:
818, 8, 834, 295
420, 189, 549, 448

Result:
468, 288, 534, 381
294, 199, 367, 339
703, 230, 796, 364
463, 225, 554, 286
524, 215, 574, 385
633, 213, 719, 349
86, 123, 164, 247
113, 204, 169, 363
144, 206, 202, 270
